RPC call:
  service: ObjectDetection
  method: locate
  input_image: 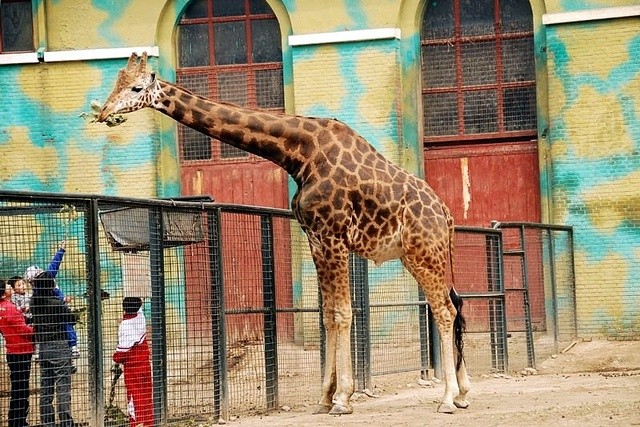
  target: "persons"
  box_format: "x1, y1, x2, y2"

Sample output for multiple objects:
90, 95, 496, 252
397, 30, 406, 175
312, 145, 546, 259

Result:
29, 272, 79, 427
112, 298, 155, 427
24, 241, 81, 359
7, 275, 40, 356
0, 279, 34, 427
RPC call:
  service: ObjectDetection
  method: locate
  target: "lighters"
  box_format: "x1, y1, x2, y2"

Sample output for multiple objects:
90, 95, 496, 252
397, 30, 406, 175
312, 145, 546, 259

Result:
92, 49, 471, 416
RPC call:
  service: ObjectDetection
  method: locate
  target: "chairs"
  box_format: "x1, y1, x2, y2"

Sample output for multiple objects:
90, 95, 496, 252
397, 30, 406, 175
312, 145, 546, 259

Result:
71, 346, 80, 359
